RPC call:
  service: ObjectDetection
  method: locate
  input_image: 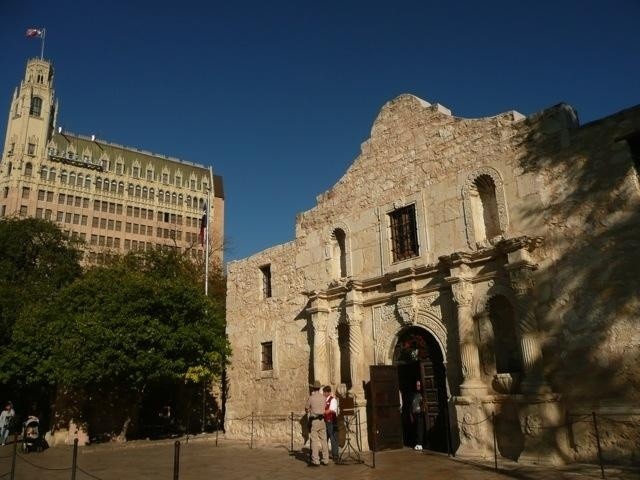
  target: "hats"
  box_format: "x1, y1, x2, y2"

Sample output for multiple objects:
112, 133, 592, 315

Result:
307, 380, 324, 390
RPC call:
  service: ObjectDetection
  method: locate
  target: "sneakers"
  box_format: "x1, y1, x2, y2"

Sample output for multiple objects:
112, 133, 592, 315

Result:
414, 445, 423, 451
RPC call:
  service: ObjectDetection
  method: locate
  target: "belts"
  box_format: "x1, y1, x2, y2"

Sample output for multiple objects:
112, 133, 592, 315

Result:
311, 416, 323, 420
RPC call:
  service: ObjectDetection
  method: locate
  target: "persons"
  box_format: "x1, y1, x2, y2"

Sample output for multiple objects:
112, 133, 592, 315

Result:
409, 380, 425, 451
27, 427, 38, 436
305, 380, 329, 466
0, 401, 16, 446
323, 386, 339, 462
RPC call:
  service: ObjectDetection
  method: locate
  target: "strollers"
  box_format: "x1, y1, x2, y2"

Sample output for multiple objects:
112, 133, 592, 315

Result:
17, 415, 46, 455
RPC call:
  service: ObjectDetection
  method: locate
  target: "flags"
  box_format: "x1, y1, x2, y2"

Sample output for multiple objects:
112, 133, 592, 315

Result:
26, 29, 44, 38
199, 198, 208, 247
